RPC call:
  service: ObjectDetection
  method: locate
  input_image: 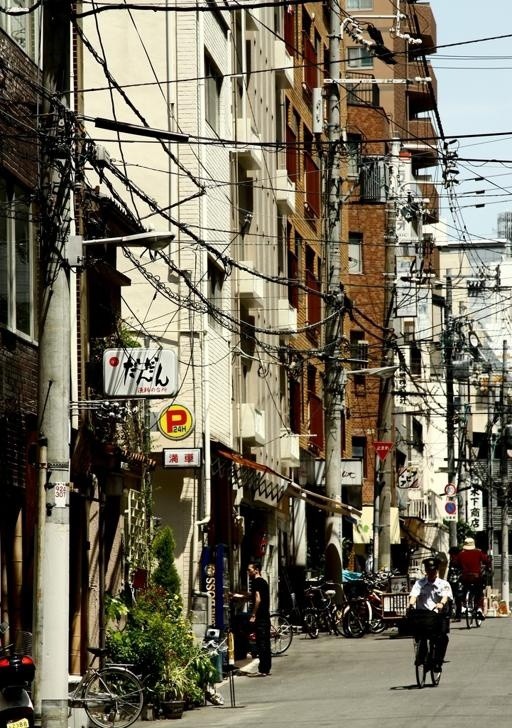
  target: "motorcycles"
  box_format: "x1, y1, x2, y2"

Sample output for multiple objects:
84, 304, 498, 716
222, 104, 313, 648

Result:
0, 621, 38, 728
223, 588, 258, 660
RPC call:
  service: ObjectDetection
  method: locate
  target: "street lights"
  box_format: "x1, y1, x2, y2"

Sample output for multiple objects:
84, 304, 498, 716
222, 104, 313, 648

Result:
319, 362, 401, 563
71, 227, 182, 436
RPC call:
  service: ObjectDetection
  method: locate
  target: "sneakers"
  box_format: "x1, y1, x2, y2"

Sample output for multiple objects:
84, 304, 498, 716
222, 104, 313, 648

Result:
477, 609, 486, 620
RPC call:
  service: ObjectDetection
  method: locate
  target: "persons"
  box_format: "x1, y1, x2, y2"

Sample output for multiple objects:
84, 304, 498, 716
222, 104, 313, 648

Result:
408, 559, 453, 673
232, 561, 271, 676
447, 537, 491, 622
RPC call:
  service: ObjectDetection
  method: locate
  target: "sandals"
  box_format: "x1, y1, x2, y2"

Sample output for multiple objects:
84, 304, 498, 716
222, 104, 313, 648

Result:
248, 670, 271, 677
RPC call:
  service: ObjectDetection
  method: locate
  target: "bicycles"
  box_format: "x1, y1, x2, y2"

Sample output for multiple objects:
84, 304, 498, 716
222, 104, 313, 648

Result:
242, 565, 494, 691
17, 627, 149, 728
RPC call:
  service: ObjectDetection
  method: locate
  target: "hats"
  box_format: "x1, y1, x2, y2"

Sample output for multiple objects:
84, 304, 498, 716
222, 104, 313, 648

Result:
423, 558, 441, 568
462, 537, 475, 551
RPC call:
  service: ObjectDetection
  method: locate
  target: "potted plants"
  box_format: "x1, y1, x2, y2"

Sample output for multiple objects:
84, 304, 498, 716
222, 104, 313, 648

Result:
102, 524, 226, 720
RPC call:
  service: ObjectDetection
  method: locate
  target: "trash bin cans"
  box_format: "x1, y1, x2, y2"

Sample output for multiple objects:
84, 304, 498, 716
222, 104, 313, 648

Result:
202, 637, 228, 683
